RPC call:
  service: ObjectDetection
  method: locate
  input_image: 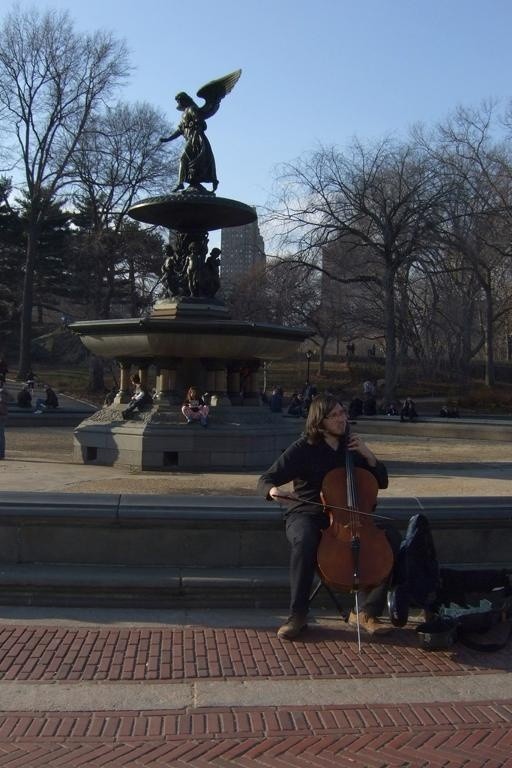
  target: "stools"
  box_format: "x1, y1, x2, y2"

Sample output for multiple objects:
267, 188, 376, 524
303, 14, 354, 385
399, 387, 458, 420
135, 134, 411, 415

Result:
306, 578, 349, 622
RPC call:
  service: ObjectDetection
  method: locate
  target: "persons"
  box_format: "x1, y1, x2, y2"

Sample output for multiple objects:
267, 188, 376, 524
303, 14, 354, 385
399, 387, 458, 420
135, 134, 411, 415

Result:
181, 386, 209, 426
256, 393, 390, 639
158, 240, 221, 297
346, 341, 355, 356
439, 401, 460, 418
0, 360, 58, 459
104, 374, 156, 418
160, 69, 243, 193
271, 381, 418, 423
367, 344, 376, 356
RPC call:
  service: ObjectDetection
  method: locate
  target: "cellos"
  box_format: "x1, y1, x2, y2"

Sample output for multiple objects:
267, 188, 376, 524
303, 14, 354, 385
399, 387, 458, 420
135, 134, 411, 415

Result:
316, 399, 394, 591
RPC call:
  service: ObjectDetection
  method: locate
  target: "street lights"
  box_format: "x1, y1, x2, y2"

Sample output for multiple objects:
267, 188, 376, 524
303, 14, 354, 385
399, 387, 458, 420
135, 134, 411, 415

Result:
305, 350, 313, 390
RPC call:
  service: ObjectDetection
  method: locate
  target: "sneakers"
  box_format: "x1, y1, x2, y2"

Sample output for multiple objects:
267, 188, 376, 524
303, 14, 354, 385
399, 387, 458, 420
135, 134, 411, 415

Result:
278, 614, 307, 638
348, 607, 392, 635
